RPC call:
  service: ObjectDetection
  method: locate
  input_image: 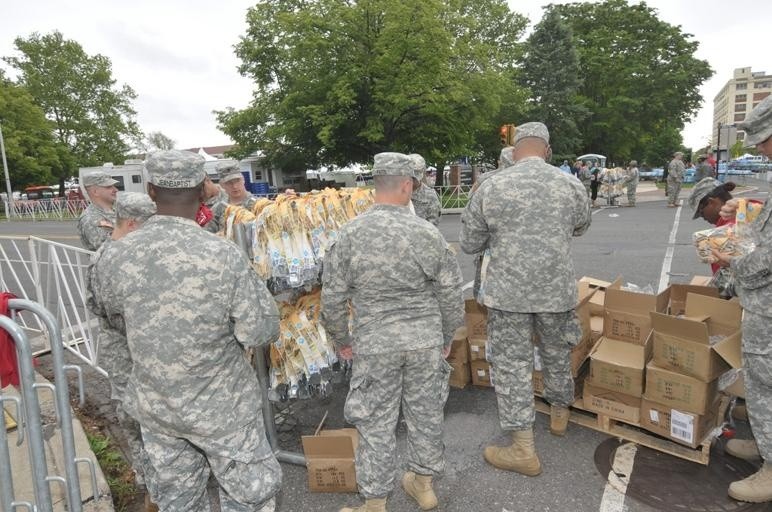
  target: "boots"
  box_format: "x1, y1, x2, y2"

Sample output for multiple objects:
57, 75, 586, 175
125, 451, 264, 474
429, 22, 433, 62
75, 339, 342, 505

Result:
483, 427, 543, 477
728, 461, 772, 503
402, 470, 438, 510
550, 404, 570, 436
337, 496, 387, 512
724, 438, 762, 461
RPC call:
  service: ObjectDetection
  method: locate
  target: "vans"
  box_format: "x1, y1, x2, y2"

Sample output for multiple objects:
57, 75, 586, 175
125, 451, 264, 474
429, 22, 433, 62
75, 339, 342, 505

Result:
744, 156, 762, 163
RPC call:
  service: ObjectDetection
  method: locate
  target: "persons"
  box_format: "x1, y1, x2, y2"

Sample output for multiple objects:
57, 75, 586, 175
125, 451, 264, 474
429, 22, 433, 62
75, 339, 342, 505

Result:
319, 152, 466, 511
689, 178, 766, 423
76, 150, 297, 512
463, 146, 516, 301
458, 122, 592, 476
406, 153, 441, 230
558, 156, 638, 209
661, 152, 715, 207
712, 95, 772, 504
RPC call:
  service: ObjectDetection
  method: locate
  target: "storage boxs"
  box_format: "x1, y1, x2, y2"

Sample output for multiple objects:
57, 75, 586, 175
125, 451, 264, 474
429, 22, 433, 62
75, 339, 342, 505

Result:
300, 428, 360, 494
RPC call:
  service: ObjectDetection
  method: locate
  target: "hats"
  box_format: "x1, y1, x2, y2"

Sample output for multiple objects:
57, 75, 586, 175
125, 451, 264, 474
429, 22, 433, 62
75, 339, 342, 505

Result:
512, 121, 552, 162
500, 146, 514, 170
739, 94, 772, 148
408, 154, 426, 180
371, 152, 421, 191
82, 149, 243, 224
688, 176, 725, 220
